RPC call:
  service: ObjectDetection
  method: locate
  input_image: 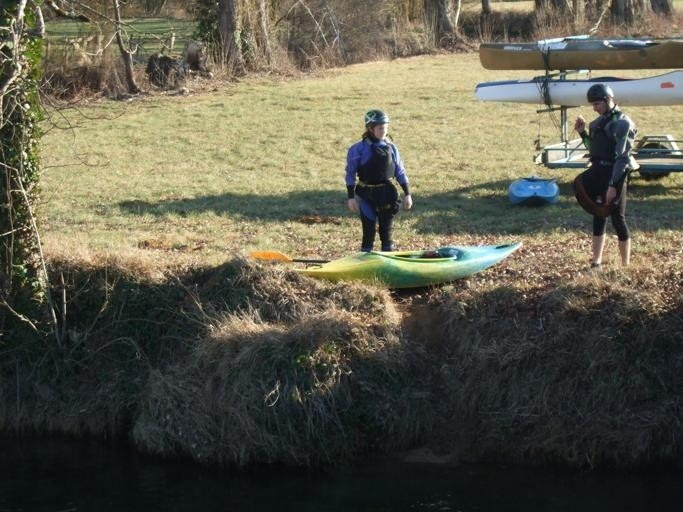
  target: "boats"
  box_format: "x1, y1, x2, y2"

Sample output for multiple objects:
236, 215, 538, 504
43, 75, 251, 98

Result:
287, 235, 524, 292
474, 35, 682, 204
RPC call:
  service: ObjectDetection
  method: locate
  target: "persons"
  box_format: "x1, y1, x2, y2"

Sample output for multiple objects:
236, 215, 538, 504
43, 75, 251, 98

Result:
574, 84, 640, 263
345, 109, 412, 253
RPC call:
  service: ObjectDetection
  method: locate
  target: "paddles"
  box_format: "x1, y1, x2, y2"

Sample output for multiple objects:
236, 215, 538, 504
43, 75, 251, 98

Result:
247, 249, 332, 264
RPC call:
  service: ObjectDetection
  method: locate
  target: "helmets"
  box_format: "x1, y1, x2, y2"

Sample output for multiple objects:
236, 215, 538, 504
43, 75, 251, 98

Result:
365, 109, 390, 129
588, 84, 614, 103
